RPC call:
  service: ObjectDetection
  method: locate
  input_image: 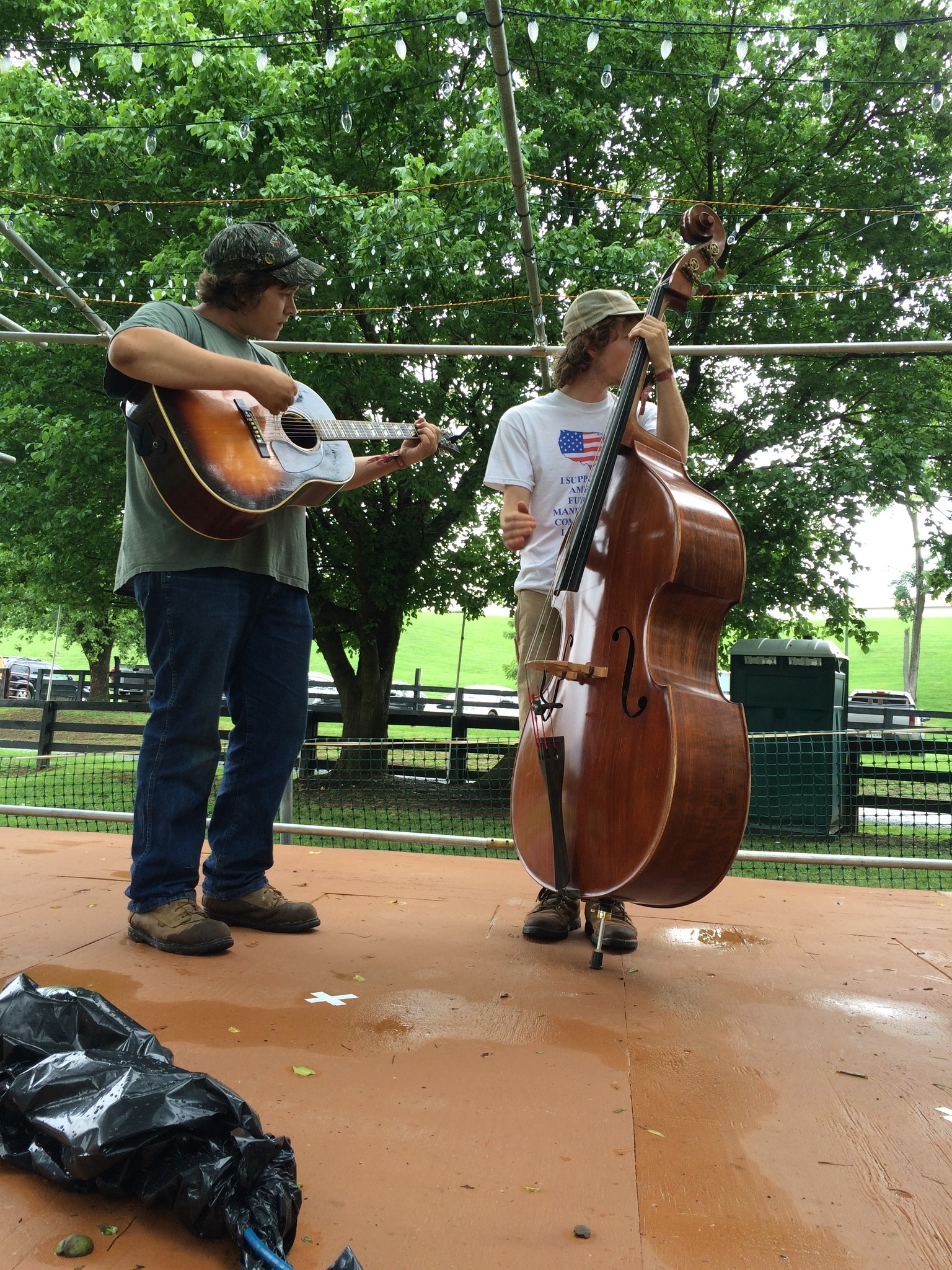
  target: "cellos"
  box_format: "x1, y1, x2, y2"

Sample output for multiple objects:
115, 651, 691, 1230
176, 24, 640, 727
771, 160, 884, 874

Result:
510, 204, 752, 969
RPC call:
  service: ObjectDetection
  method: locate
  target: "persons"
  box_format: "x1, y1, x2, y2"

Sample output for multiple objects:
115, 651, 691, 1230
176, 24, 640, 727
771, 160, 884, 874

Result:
105, 224, 443, 953
479, 290, 692, 950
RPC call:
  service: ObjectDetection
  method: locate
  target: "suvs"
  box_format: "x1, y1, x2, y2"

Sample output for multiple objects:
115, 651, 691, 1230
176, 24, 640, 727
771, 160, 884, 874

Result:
2, 656, 90, 701
109, 664, 156, 703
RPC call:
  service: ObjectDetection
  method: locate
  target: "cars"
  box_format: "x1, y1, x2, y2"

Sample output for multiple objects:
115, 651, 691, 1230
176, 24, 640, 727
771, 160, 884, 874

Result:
845, 689, 931, 758
422, 684, 519, 717
388, 681, 428, 712
307, 671, 342, 713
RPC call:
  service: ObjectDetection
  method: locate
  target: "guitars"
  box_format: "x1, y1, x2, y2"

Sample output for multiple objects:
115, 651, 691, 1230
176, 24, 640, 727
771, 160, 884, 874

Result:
124, 378, 460, 541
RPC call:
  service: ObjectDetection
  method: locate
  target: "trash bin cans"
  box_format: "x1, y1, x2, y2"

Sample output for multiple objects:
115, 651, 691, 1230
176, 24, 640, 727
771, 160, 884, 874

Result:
727, 638, 846, 843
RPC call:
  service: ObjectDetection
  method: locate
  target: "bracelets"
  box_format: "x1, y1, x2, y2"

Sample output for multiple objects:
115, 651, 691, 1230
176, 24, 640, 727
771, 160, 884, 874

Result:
638, 365, 673, 420
364, 450, 407, 470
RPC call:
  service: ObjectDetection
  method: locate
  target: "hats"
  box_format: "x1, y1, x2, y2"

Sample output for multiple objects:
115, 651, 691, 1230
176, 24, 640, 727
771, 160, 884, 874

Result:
564, 289, 666, 346
206, 221, 327, 290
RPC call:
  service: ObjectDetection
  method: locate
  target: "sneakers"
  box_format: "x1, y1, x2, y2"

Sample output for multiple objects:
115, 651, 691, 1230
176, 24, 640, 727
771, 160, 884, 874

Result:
523, 887, 581, 939
201, 883, 321, 929
127, 899, 234, 955
584, 901, 638, 949
